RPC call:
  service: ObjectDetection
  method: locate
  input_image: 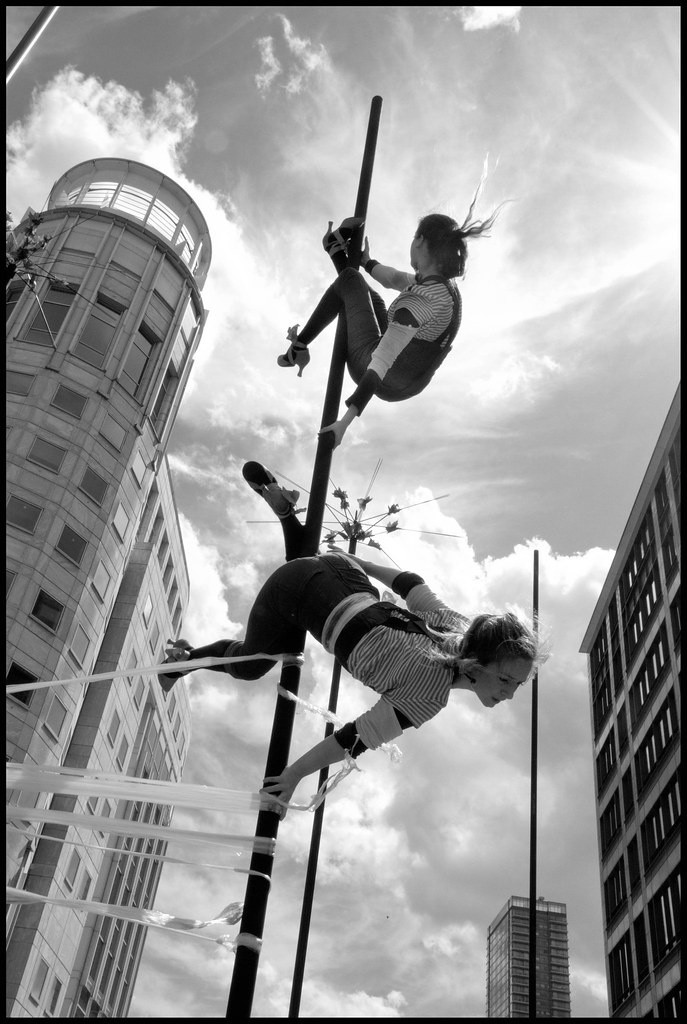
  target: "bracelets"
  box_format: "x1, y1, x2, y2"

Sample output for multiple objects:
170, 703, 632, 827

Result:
365, 259, 380, 275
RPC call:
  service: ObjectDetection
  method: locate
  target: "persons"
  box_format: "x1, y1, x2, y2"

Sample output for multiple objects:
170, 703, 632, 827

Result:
157, 460, 550, 821
277, 154, 516, 453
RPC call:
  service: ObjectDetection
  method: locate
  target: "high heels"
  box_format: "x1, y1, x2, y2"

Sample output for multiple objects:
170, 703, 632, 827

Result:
322, 216, 366, 258
277, 324, 310, 377
158, 638, 195, 693
242, 461, 299, 520
